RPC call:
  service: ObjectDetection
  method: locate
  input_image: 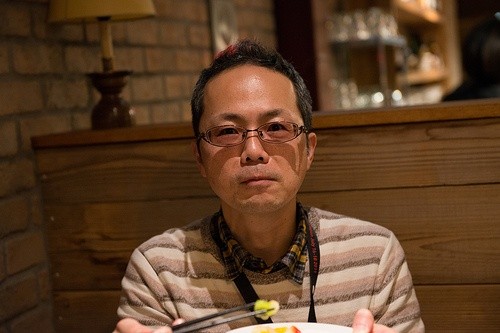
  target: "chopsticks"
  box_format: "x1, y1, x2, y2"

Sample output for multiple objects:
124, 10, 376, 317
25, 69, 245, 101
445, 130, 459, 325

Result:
171, 298, 274, 333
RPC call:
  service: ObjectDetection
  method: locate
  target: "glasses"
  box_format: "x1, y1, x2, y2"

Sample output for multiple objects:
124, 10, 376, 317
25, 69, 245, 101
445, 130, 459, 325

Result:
198, 122, 307, 147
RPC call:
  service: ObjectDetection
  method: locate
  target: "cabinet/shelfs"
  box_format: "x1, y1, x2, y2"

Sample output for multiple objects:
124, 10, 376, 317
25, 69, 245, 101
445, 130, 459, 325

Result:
334, 1, 451, 110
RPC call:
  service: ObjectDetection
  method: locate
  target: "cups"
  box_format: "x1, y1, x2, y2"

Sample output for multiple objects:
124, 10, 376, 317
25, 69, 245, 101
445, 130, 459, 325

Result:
326, 1, 448, 111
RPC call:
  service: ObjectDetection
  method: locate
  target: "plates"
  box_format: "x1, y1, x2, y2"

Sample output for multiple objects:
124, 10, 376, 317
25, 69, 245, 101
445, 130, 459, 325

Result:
223, 321, 357, 333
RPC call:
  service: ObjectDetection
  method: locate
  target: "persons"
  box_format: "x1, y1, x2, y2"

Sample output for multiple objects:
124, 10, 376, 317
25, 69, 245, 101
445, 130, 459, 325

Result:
442, 18, 500, 101
111, 41, 425, 333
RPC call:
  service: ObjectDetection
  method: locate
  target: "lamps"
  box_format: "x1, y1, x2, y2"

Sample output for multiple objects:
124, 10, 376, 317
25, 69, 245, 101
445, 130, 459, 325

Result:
45, 0, 158, 126
